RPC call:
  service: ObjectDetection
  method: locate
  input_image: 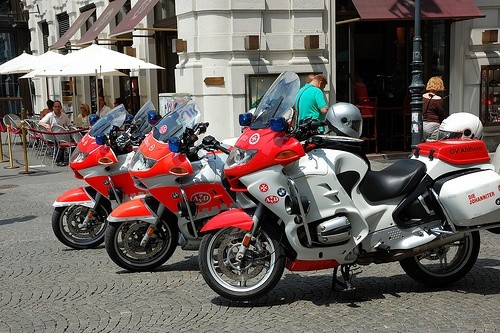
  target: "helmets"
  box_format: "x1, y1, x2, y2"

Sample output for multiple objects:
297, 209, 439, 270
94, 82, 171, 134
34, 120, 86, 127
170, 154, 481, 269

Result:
326, 102, 362, 137
438, 112, 483, 140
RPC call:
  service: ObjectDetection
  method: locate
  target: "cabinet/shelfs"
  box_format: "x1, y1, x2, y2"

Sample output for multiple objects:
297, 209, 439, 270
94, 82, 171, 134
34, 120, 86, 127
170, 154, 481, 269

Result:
480, 65, 500, 126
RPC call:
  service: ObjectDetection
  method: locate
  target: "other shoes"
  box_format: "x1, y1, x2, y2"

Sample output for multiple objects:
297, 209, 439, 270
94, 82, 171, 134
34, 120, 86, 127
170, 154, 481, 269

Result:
57, 161, 66, 166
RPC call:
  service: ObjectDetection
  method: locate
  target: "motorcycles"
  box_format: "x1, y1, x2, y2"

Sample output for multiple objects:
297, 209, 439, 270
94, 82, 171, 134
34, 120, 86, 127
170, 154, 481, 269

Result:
199, 71, 499, 301
52, 103, 163, 250
105, 100, 294, 272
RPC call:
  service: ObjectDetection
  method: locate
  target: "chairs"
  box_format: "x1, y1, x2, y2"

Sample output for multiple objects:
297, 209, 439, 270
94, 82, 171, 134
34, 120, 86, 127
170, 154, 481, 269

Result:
404, 96, 412, 151
4, 114, 85, 170
356, 97, 378, 154
